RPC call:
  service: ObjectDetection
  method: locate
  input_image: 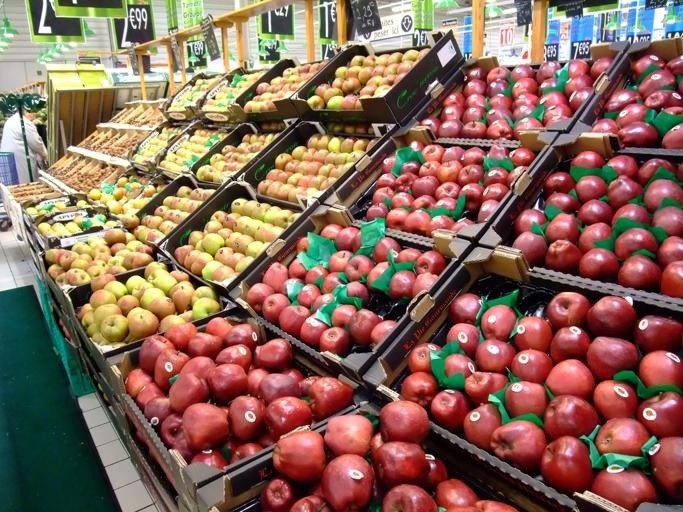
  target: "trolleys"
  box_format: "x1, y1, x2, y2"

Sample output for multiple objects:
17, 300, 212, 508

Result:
0, 152, 20, 232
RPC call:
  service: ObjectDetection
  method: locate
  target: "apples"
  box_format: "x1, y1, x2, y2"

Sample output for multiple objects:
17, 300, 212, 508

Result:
25, 47, 683, 512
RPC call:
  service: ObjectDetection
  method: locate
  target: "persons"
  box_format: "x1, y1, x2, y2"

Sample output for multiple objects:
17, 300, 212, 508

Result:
0, 109, 48, 184
